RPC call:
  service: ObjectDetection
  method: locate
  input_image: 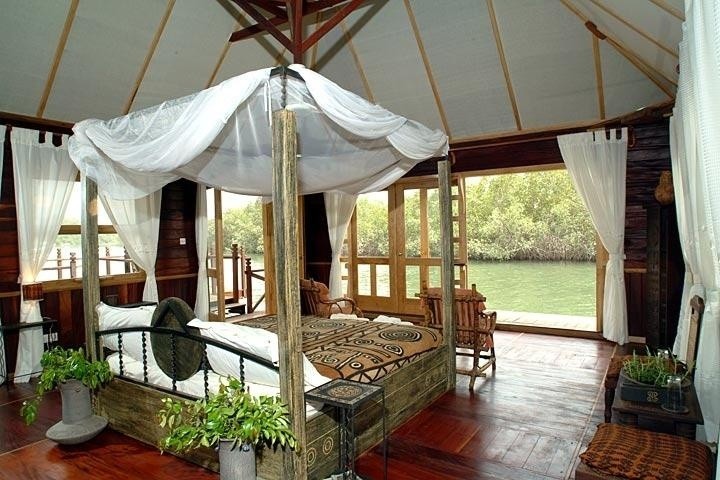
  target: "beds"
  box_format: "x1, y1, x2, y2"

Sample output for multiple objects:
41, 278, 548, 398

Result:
81, 65, 456, 480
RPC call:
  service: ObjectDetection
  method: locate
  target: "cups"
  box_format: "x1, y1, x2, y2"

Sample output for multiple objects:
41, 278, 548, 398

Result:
658, 348, 668, 363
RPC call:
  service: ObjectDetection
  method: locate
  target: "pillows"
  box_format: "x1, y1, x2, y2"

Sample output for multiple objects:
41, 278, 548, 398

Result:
185, 319, 332, 386
94, 301, 157, 367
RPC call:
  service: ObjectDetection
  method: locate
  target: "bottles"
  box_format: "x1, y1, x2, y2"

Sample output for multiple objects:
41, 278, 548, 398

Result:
666, 376, 682, 411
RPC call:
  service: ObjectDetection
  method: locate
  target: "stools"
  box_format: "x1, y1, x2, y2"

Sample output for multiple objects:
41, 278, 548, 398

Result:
604, 353, 670, 423
575, 423, 714, 480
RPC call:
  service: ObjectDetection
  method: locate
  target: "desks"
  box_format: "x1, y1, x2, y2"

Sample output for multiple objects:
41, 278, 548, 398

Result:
611, 366, 704, 440
304, 377, 385, 480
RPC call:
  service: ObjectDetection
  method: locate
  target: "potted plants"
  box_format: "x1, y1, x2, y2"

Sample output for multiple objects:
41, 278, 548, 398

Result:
16, 345, 114, 445
155, 374, 300, 480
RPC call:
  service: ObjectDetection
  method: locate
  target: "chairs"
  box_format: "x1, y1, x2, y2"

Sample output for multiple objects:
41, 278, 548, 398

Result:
300, 277, 364, 318
415, 280, 496, 390
